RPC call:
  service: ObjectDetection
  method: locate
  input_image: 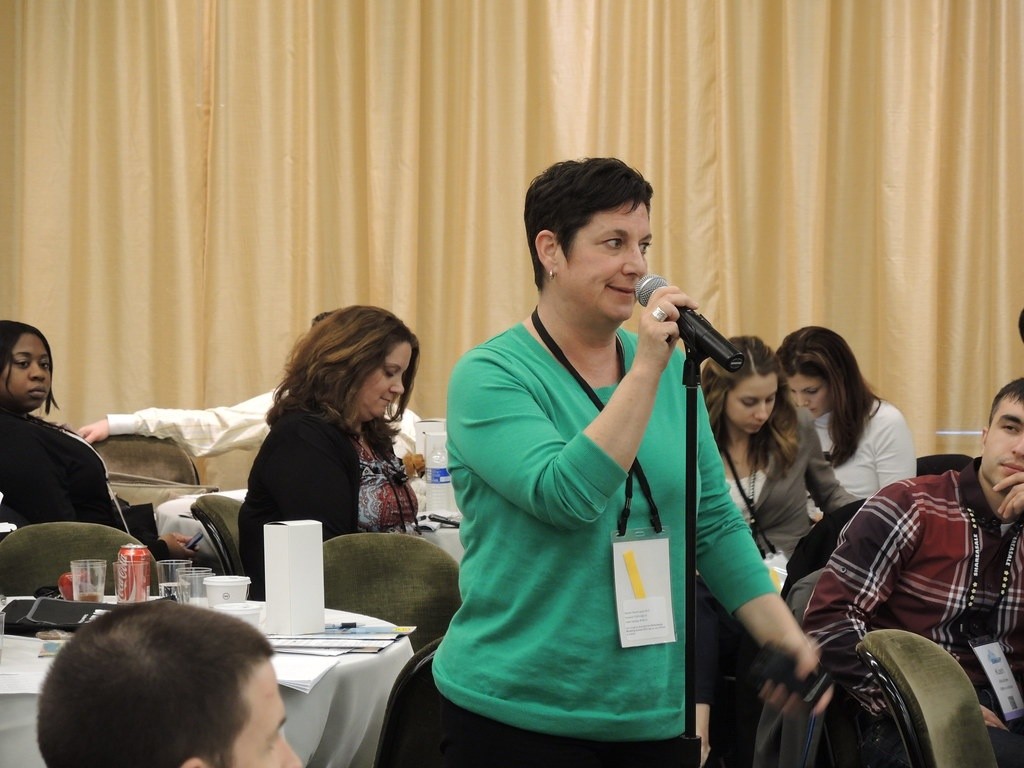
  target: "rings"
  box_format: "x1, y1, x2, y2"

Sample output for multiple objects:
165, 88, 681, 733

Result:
650, 306, 668, 322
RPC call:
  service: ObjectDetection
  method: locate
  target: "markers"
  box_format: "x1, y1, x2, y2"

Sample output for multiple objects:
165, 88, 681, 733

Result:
413, 515, 426, 523
428, 514, 461, 528
325, 622, 356, 629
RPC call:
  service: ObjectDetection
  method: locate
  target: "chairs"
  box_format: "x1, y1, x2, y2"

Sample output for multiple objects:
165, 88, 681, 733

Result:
856, 629, 998, 768
0, 434, 462, 768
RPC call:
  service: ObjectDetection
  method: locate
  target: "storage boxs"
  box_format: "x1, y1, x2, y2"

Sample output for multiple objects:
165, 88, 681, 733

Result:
263, 520, 325, 634
415, 418, 447, 457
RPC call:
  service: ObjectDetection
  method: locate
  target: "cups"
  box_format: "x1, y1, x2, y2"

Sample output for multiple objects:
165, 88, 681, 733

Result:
156, 560, 192, 605
214, 604, 262, 629
113, 561, 148, 603
202, 576, 252, 608
174, 567, 215, 608
70, 560, 107, 603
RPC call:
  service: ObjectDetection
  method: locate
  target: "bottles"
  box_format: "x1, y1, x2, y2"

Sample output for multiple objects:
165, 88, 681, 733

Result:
424, 441, 454, 512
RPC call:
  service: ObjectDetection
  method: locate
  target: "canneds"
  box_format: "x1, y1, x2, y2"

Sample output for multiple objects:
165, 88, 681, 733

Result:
117, 543, 151, 604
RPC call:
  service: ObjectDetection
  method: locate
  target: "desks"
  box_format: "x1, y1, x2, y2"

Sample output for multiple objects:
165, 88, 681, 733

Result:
0, 596, 414, 768
155, 489, 463, 576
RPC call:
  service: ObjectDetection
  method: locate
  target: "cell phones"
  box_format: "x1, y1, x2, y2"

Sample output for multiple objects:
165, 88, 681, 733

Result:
184, 531, 204, 549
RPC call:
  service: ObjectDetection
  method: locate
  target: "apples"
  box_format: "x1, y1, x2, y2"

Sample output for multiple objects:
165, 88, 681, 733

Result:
58, 571, 87, 601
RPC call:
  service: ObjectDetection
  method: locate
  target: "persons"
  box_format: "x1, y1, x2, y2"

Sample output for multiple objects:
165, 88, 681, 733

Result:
38, 603, 302, 768
772, 326, 917, 525
702, 335, 859, 595
77, 312, 422, 575
429, 156, 834, 768
239, 307, 418, 603
0, 321, 200, 560
801, 377, 1024, 768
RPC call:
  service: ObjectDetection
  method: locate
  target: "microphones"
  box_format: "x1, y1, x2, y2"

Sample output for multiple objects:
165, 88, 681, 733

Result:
634, 274, 746, 373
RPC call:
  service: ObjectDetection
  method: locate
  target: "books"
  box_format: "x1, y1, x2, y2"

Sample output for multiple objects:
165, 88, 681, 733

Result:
247, 625, 418, 692
414, 508, 451, 532
